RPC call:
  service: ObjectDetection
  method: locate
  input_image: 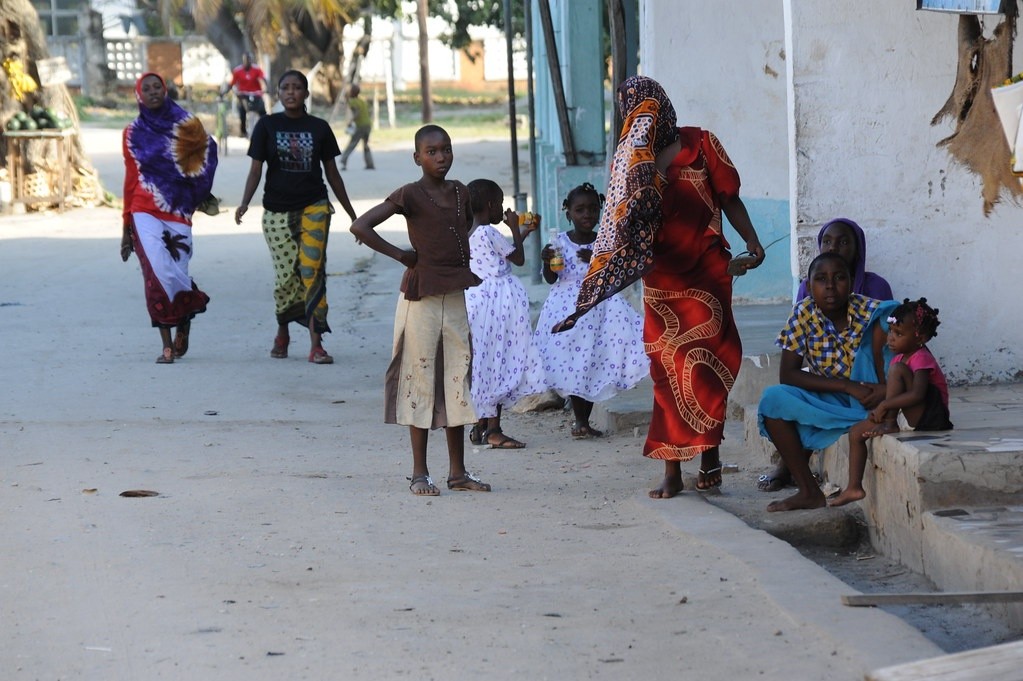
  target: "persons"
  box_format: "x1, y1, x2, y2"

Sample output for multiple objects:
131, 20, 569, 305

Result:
550, 73, 767, 500
120, 71, 221, 364
532, 180, 652, 440
339, 83, 377, 171
218, 51, 274, 138
234, 68, 363, 366
756, 216, 956, 513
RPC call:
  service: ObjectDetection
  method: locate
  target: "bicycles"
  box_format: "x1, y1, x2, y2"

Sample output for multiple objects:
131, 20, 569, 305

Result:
200, 83, 234, 157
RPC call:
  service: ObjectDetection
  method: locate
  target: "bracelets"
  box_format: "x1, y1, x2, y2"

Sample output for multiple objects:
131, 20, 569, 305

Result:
347, 209, 354, 214
348, 123, 550, 498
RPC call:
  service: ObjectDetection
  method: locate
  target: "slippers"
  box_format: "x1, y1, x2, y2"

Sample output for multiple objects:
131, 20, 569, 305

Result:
695, 461, 722, 491
756, 476, 788, 491
727, 251, 758, 276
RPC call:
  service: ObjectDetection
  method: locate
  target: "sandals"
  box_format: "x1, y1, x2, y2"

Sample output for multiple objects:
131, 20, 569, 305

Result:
405, 476, 440, 495
481, 427, 526, 449
156, 345, 174, 363
446, 472, 491, 492
173, 321, 191, 356
469, 425, 487, 445
572, 422, 603, 436
308, 346, 333, 363
271, 335, 290, 358
